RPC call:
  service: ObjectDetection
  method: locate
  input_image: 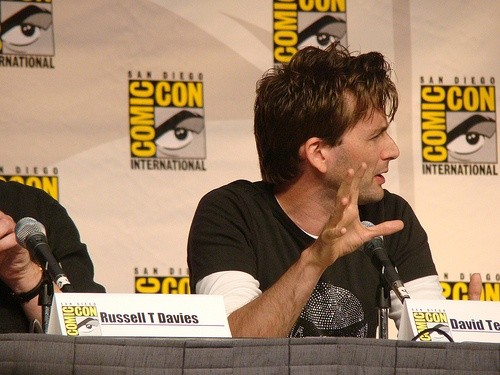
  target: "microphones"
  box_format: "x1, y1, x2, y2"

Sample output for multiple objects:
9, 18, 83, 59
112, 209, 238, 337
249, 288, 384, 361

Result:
357, 220, 410, 303
14, 216, 75, 292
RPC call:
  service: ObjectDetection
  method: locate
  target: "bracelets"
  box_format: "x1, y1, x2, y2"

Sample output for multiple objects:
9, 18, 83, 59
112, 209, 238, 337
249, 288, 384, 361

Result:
6, 269, 47, 303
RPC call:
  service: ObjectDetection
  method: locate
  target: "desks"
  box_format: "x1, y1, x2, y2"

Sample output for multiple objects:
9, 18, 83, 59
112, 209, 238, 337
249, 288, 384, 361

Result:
0, 333, 500, 375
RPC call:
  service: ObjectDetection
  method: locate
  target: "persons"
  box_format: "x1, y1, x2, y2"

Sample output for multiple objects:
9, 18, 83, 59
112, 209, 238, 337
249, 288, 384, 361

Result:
0, 178, 108, 332
185, 40, 483, 340
